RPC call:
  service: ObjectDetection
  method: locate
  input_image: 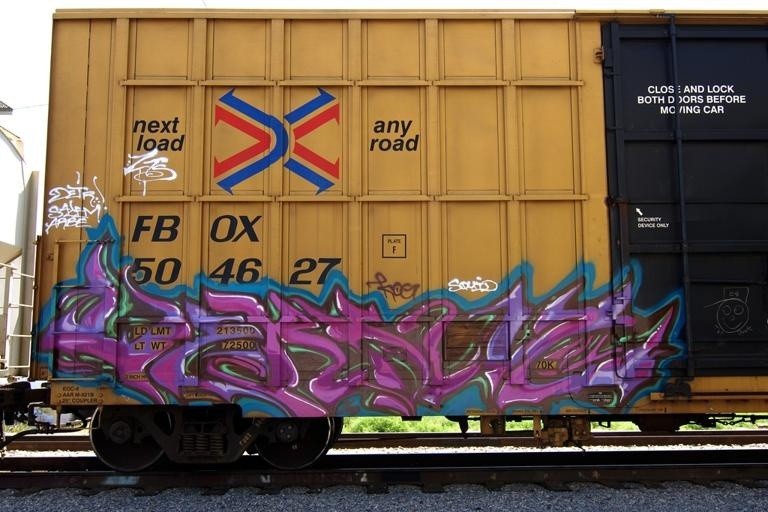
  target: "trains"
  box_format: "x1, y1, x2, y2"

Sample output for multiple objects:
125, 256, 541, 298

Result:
1, 9, 767, 475
